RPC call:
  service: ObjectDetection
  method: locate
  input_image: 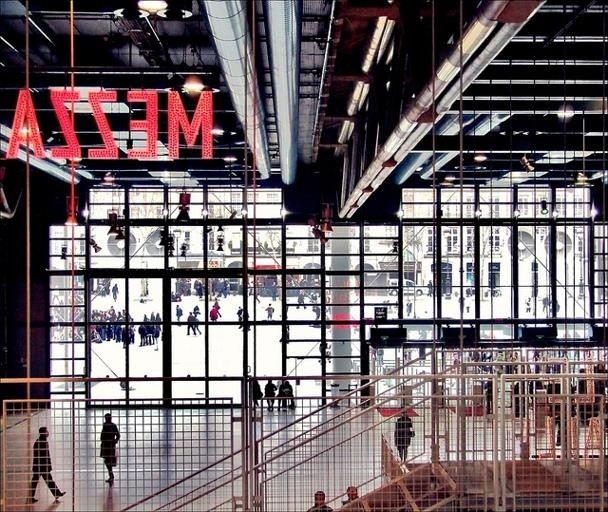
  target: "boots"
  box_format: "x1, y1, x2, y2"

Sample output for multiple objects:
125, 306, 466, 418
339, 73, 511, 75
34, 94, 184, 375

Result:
105, 471, 114, 487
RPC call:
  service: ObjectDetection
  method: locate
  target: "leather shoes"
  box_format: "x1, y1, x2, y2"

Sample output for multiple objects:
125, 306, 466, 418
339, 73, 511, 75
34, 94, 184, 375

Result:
26, 497, 38, 502
55, 491, 66, 500
253, 404, 295, 411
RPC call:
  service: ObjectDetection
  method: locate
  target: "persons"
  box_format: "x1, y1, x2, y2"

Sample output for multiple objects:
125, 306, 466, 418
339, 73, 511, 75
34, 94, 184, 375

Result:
278, 381, 287, 412
264, 303, 275, 319
427, 279, 433, 296
542, 296, 561, 319
193, 305, 200, 318
99, 413, 121, 483
306, 490, 333, 512
214, 297, 220, 310
418, 348, 427, 366
265, 378, 277, 410
90, 305, 164, 352
407, 299, 412, 316
236, 306, 245, 329
175, 304, 183, 321
341, 486, 360, 505
284, 276, 334, 365
394, 409, 415, 462
187, 312, 197, 335
112, 283, 119, 301
210, 306, 221, 322
370, 347, 386, 366
525, 297, 532, 312
271, 281, 278, 301
242, 308, 252, 331
281, 380, 295, 410
193, 314, 202, 335
471, 347, 608, 446
252, 379, 263, 408
23, 426, 66, 503
171, 277, 231, 305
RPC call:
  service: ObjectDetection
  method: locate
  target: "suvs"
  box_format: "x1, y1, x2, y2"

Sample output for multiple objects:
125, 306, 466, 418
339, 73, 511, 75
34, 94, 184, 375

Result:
388, 279, 431, 296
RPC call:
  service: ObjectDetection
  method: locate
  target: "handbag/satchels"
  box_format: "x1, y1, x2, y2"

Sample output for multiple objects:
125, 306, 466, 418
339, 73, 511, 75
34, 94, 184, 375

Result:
112, 457, 117, 466
409, 430, 415, 437
